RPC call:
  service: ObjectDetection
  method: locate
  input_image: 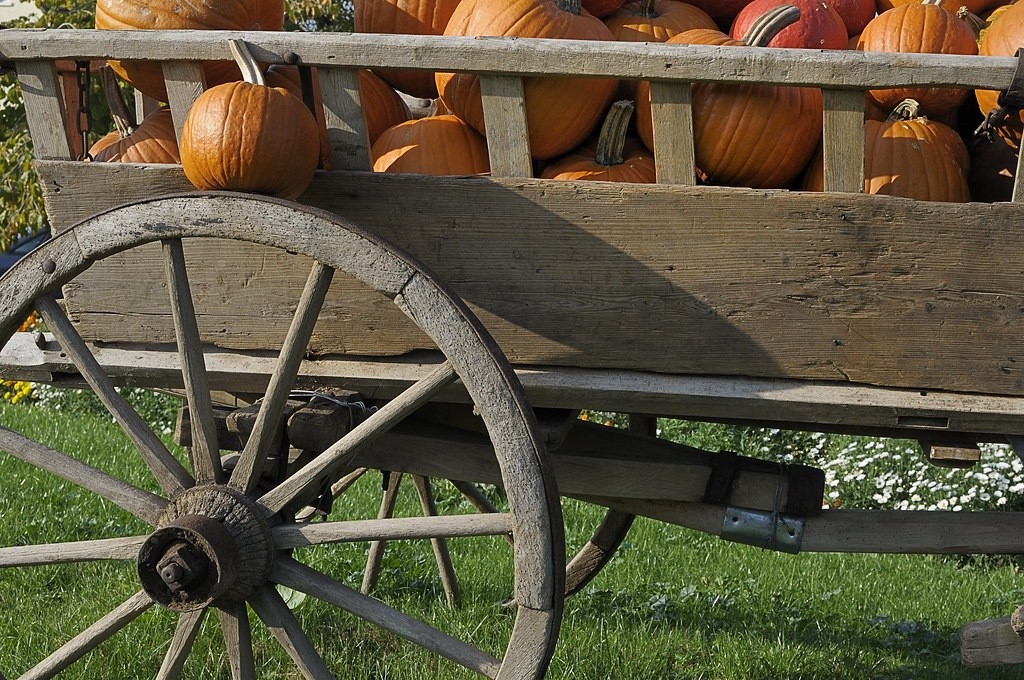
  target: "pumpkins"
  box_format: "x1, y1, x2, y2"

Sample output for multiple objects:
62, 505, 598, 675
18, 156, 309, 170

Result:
77, 0, 1024, 203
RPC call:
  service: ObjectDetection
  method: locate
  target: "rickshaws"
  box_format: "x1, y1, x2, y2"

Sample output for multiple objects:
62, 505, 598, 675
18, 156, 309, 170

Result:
0, 0, 1024, 680
